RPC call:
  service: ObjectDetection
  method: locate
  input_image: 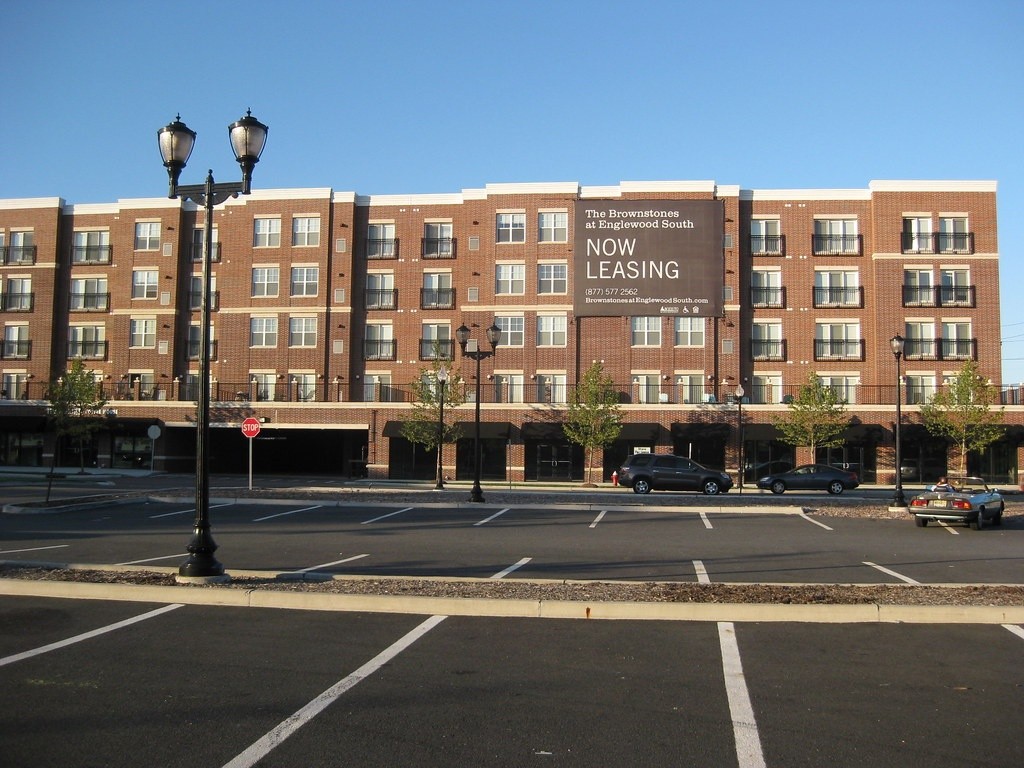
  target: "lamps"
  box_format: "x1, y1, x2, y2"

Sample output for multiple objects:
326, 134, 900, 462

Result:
486, 375, 492, 380
104, 375, 110, 379
356, 375, 360, 379
663, 375, 667, 380
976, 375, 981, 380
277, 374, 281, 379
317, 374, 322, 379
120, 374, 125, 380
899, 375, 903, 380
27, 373, 32, 378
744, 378, 748, 381
707, 375, 712, 379
530, 375, 534, 379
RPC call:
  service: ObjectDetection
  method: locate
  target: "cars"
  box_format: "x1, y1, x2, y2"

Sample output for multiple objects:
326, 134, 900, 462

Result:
755, 463, 860, 495
901, 457, 916, 480
926, 458, 945, 481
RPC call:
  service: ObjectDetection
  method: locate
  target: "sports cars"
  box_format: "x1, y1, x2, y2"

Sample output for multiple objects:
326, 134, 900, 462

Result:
907, 476, 1005, 530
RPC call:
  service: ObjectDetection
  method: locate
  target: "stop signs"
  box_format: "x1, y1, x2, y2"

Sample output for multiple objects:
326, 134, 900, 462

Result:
241, 417, 262, 438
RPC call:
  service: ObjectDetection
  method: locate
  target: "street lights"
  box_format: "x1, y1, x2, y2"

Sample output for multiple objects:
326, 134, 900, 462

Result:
455, 323, 502, 504
889, 332, 909, 507
153, 105, 270, 581
736, 382, 745, 496
434, 363, 451, 490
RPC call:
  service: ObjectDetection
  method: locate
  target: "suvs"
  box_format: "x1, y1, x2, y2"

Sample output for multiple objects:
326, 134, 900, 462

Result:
621, 452, 734, 495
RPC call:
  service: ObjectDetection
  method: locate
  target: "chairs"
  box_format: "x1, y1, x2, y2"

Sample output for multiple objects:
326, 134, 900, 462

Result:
935, 486, 948, 492
961, 488, 973, 493
806, 468, 812, 473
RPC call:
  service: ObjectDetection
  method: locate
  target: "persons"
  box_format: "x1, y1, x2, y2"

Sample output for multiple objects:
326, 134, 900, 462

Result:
35, 440, 44, 467
931, 477, 956, 493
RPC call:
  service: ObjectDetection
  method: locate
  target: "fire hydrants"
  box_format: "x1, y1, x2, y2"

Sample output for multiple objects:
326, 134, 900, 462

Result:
611, 471, 619, 487
